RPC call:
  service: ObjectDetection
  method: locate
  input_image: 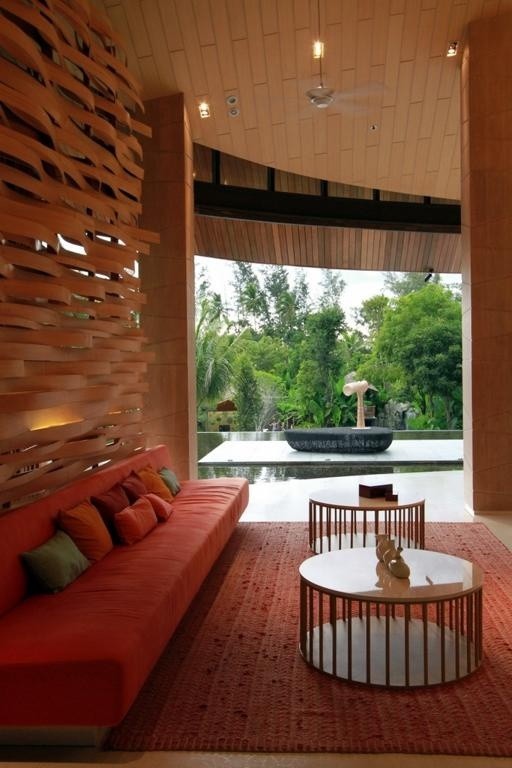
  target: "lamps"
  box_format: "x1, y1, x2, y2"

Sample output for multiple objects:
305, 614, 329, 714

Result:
304, 38, 336, 107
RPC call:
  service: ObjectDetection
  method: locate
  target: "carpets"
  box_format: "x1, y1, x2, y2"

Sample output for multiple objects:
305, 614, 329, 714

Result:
103, 519, 512, 756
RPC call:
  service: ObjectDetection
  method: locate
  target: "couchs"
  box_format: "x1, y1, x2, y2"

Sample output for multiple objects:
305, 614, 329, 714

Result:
0, 441, 252, 746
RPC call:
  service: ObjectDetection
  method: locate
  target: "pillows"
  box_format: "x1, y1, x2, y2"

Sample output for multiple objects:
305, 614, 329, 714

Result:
18, 461, 183, 596
